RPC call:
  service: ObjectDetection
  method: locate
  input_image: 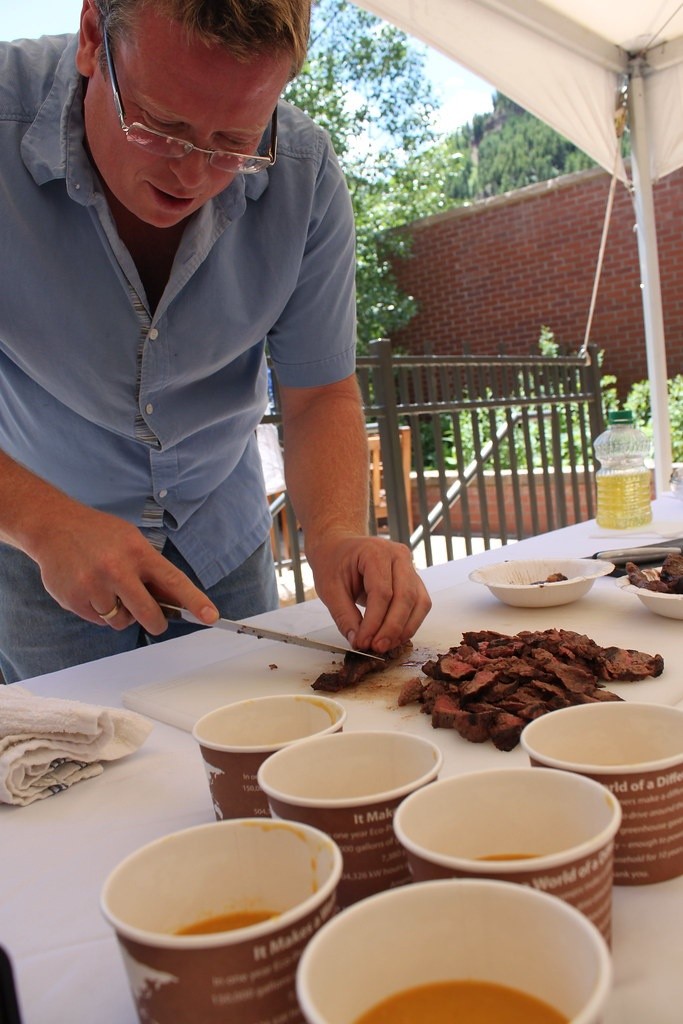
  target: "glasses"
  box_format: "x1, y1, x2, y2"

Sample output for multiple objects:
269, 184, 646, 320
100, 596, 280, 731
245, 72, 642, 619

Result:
103, 27, 278, 174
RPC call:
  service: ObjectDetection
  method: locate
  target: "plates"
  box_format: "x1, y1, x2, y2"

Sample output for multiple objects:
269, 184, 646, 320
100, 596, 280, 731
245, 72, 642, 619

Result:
614, 567, 683, 620
467, 558, 616, 608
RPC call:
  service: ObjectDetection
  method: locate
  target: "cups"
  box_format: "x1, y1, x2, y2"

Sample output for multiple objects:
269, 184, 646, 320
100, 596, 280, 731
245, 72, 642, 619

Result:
521, 701, 683, 886
393, 767, 621, 954
192, 694, 347, 822
100, 817, 345, 1024
294, 877, 614, 1024
257, 731, 443, 908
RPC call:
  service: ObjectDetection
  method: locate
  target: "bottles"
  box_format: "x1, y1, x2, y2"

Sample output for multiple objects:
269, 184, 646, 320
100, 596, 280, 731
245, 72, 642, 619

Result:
592, 411, 655, 529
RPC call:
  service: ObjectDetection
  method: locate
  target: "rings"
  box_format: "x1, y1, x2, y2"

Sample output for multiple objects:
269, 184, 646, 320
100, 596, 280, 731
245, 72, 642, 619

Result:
97, 597, 121, 619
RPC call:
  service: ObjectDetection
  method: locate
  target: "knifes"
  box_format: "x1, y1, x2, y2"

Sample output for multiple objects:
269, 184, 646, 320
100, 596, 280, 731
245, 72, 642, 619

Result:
157, 602, 385, 663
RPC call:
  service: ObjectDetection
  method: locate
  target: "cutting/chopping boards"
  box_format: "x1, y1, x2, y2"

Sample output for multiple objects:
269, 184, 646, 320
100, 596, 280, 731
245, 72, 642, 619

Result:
122, 575, 683, 785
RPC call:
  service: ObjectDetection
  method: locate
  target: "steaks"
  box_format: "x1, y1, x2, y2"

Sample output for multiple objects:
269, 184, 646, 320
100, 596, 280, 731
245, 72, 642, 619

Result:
626, 551, 683, 594
310, 629, 663, 751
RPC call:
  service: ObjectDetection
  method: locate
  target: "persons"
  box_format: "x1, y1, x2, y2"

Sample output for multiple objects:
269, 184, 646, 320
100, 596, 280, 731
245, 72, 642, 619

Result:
0, 1, 433, 689
